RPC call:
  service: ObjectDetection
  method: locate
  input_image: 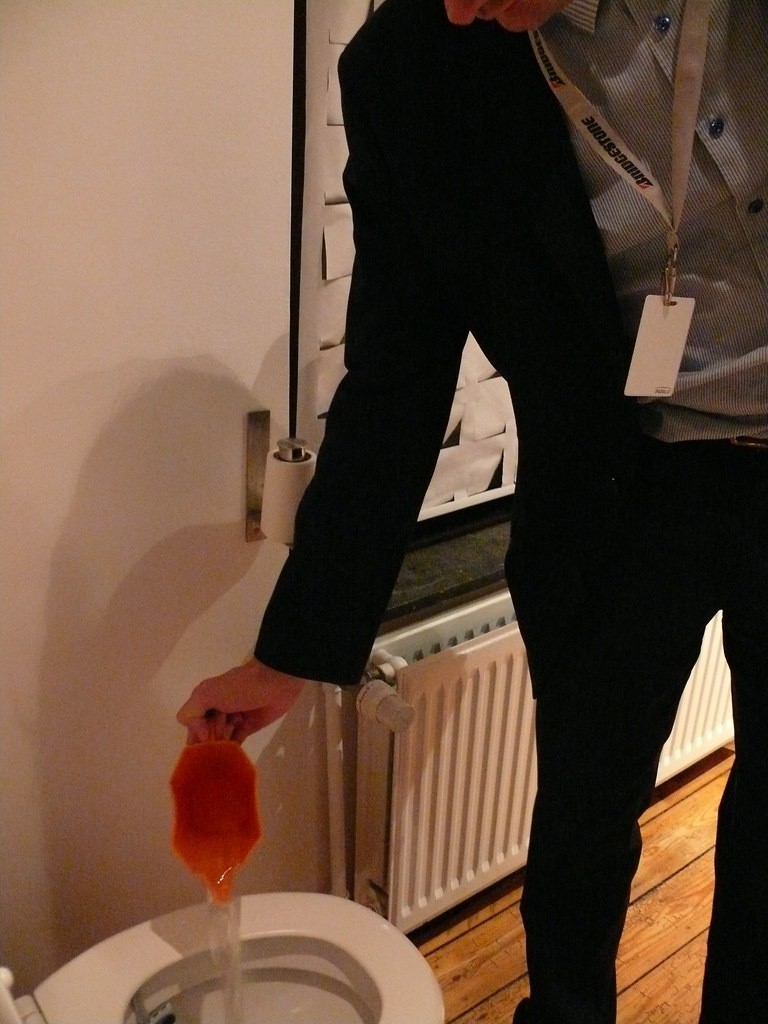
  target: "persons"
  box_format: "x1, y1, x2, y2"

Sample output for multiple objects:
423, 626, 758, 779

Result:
174, 0, 768, 1024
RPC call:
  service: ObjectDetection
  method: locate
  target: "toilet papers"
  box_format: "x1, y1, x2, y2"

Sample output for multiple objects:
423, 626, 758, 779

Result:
259, 446, 317, 544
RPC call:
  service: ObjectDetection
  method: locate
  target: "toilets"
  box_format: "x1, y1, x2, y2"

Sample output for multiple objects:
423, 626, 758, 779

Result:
0, 877, 446, 1024
